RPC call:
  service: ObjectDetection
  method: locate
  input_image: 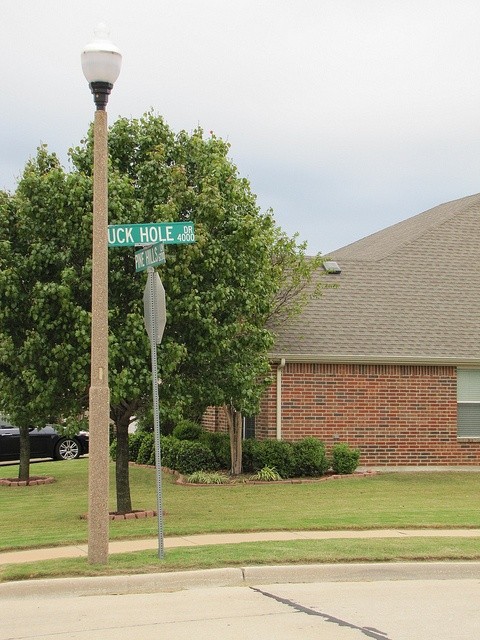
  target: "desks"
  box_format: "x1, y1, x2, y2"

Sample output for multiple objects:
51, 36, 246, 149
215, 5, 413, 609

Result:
78, 48, 122, 567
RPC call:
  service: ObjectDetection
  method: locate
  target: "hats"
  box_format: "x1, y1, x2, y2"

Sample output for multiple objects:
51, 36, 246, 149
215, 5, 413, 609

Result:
0, 414, 89, 461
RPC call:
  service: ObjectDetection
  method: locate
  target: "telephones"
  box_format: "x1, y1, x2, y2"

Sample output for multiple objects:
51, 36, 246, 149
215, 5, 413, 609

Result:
134, 241, 168, 274
108, 222, 195, 249
